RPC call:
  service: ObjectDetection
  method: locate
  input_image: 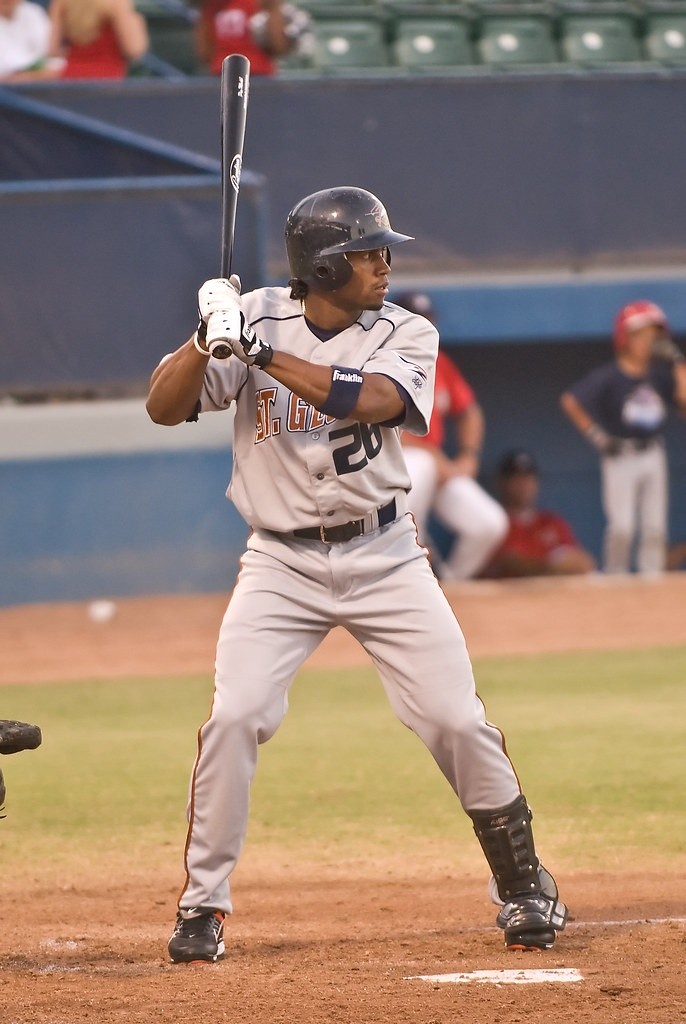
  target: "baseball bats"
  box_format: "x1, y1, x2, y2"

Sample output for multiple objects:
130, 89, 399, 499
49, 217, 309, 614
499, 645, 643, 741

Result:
207, 51, 262, 357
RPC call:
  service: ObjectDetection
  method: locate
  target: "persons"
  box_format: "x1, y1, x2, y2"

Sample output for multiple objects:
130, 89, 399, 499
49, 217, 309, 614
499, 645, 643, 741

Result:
0, 0, 303, 82
146, 187, 567, 965
388, 291, 686, 575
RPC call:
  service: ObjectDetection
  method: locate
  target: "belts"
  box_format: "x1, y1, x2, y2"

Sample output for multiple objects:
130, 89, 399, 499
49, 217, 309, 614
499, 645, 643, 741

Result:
626, 437, 660, 451
293, 497, 396, 544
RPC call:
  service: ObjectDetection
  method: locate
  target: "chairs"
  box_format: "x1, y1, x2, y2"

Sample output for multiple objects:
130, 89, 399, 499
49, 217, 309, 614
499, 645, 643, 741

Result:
559, 13, 641, 63
643, 13, 686, 58
309, 21, 385, 68
393, 16, 473, 66
476, 15, 559, 65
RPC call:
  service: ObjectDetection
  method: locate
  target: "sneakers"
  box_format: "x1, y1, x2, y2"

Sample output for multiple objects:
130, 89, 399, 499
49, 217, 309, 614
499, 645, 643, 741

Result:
496, 894, 569, 951
168, 907, 226, 962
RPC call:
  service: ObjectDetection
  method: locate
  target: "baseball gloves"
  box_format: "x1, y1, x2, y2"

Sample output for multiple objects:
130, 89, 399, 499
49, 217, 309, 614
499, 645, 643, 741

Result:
0, 716, 46, 821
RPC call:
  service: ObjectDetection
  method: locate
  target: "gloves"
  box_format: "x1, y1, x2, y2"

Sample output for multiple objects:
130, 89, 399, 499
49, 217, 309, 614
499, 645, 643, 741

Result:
205, 311, 274, 369
197, 274, 242, 340
585, 424, 617, 454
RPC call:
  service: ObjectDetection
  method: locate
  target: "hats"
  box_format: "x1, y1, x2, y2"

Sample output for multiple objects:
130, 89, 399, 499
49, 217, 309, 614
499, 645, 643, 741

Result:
501, 449, 538, 476
391, 292, 435, 323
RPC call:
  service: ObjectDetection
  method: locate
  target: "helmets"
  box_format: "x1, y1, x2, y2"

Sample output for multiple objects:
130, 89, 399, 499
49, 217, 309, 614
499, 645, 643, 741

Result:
614, 301, 670, 359
284, 185, 415, 292
